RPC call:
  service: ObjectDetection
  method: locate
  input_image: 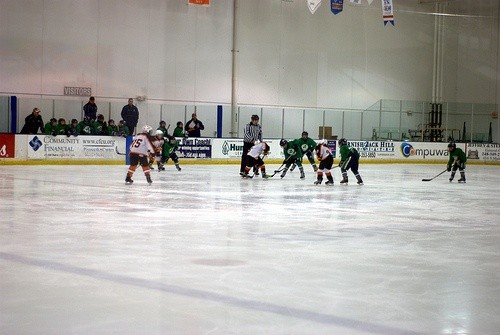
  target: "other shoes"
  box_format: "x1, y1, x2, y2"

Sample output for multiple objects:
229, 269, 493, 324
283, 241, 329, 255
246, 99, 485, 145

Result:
254, 172, 260, 177
239, 172, 249, 175
280, 171, 286, 179
300, 173, 305, 180
262, 174, 269, 180
241, 173, 248, 178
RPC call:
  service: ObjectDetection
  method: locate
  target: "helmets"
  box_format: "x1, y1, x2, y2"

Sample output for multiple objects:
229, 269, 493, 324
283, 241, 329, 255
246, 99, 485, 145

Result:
279, 139, 287, 146
302, 131, 308, 137
448, 142, 456, 152
338, 138, 348, 147
142, 122, 183, 144
50, 114, 127, 126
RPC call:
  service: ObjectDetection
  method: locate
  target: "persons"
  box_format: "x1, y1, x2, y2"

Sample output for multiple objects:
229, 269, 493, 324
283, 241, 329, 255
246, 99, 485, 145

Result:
185, 113, 204, 137
338, 138, 364, 185
44, 96, 185, 171
19, 107, 45, 133
125, 125, 155, 185
446, 143, 467, 183
239, 115, 334, 186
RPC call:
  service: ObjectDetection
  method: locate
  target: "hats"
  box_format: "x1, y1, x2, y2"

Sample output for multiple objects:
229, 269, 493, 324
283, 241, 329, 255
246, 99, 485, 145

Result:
252, 114, 259, 121
33, 108, 41, 113
90, 96, 95, 100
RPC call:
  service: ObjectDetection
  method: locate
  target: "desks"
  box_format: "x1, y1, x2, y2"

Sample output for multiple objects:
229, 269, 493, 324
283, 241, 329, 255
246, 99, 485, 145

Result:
424, 127, 461, 143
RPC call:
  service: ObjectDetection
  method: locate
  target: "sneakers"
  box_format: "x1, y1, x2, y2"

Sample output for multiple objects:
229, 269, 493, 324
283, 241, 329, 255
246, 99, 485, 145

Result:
357, 176, 364, 185
340, 176, 348, 186
124, 161, 181, 185
312, 175, 334, 186
449, 175, 466, 183
313, 164, 318, 174
289, 164, 296, 173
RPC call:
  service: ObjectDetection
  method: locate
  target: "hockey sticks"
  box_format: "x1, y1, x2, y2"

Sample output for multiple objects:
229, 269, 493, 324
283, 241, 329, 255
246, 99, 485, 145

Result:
273, 163, 292, 173
315, 165, 339, 172
116, 146, 131, 156
246, 154, 266, 178
421, 164, 455, 182
161, 123, 171, 138
265, 149, 297, 177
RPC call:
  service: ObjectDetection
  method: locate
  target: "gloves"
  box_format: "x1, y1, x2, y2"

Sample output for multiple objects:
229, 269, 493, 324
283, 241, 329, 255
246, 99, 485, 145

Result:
447, 166, 451, 172
309, 147, 313, 153
339, 160, 344, 167
292, 144, 298, 149
173, 146, 178, 152
283, 160, 288, 164
